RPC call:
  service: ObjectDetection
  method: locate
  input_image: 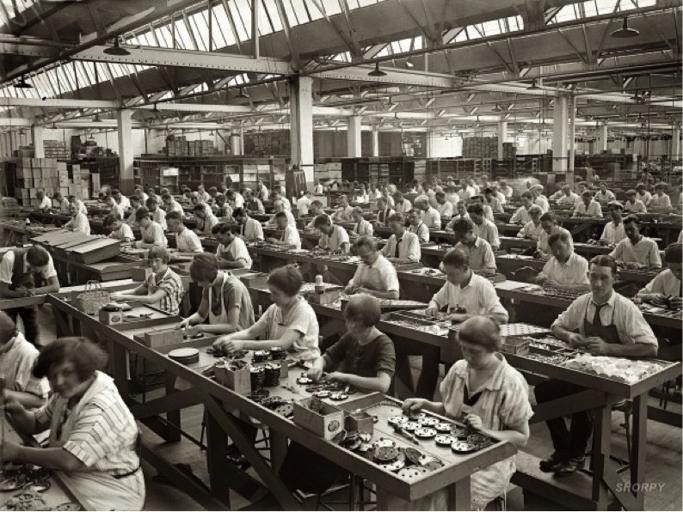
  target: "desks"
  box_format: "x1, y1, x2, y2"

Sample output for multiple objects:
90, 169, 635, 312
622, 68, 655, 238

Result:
1, 178, 682, 510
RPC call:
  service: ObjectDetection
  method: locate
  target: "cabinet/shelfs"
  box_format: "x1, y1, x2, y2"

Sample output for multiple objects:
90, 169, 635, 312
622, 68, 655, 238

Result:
61, 152, 632, 191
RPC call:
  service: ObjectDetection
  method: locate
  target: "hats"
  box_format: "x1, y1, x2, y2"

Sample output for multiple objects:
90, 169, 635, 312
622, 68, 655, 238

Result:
665, 243, 682, 263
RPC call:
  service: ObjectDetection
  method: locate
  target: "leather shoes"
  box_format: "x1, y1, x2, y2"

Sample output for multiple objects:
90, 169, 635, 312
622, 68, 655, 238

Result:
556, 454, 585, 479
540, 448, 568, 471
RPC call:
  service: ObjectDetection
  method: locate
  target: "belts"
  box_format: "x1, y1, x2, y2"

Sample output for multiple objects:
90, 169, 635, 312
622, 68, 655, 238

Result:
112, 464, 140, 478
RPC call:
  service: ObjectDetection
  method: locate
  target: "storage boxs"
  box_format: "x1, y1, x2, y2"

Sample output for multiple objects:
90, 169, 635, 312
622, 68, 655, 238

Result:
12, 155, 99, 212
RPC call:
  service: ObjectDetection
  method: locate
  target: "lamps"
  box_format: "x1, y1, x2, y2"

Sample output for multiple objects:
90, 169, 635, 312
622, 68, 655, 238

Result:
608, 0, 640, 37
14, 73, 32, 88
103, 36, 130, 56
368, 60, 387, 76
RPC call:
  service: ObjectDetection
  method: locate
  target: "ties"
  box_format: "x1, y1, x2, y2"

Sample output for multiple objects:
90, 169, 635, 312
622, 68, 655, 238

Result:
395, 239, 402, 257
592, 303, 608, 328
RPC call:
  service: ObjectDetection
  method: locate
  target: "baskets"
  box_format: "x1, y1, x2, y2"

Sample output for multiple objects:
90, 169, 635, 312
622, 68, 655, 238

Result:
76, 280, 110, 315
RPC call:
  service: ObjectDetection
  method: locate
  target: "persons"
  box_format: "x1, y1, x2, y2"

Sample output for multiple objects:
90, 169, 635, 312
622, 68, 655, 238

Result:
0, 158, 683, 510
